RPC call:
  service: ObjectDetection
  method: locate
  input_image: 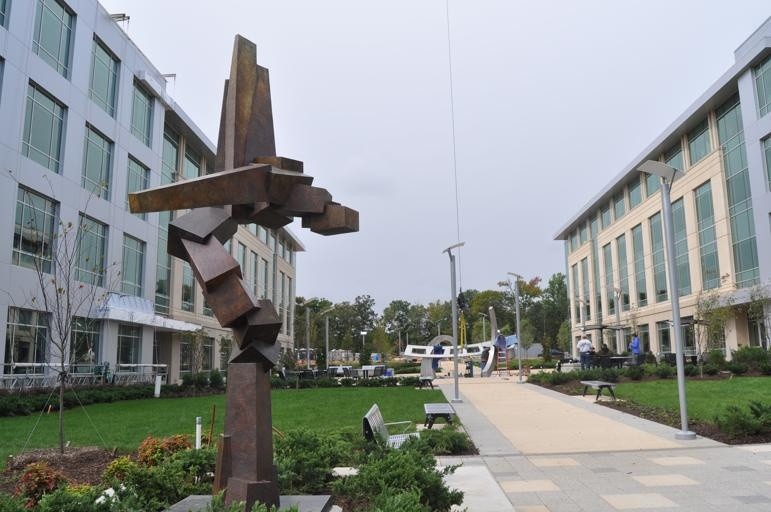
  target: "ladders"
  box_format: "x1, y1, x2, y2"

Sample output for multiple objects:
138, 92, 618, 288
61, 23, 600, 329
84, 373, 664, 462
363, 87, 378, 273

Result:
497, 346, 509, 376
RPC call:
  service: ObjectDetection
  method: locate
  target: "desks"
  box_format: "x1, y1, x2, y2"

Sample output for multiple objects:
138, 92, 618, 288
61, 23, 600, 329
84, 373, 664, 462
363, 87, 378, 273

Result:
610, 356, 632, 368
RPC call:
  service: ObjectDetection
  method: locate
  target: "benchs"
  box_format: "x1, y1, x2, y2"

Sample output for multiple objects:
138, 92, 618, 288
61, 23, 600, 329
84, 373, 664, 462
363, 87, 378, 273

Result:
424, 402, 455, 432
419, 376, 435, 389
362, 403, 421, 459
580, 380, 618, 402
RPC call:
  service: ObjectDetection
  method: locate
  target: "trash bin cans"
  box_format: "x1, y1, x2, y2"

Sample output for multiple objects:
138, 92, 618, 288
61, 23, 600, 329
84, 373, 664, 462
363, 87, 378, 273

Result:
386, 368, 395, 377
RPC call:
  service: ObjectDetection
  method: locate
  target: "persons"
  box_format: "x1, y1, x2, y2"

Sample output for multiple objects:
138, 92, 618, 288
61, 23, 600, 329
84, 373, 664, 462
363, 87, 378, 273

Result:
480, 346, 489, 369
576, 334, 591, 369
601, 343, 609, 352
629, 332, 641, 365
